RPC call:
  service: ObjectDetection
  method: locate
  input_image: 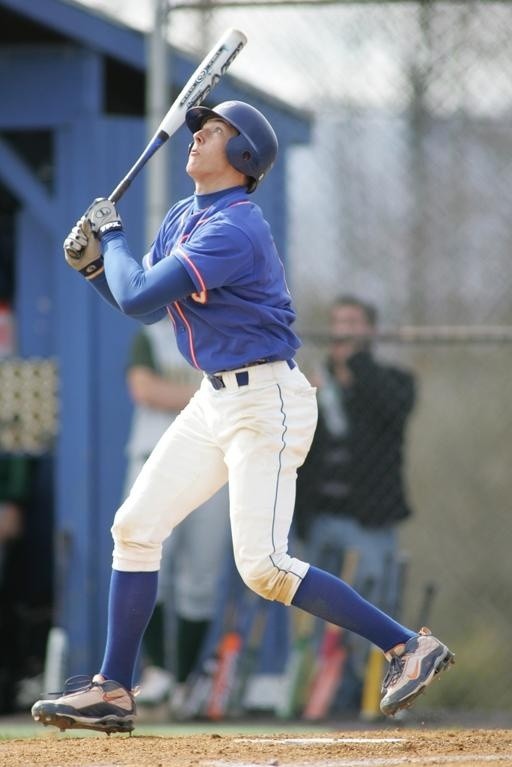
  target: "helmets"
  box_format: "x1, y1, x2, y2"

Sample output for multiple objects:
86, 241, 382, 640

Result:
186, 100, 279, 194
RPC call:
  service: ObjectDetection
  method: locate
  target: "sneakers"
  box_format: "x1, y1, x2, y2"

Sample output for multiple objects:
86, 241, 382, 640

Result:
379, 627, 456, 715
32, 675, 143, 736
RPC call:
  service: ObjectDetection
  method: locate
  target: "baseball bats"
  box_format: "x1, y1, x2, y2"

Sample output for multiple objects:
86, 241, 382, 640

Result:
108, 27, 248, 204
140, 119, 143, 125
177, 546, 438, 722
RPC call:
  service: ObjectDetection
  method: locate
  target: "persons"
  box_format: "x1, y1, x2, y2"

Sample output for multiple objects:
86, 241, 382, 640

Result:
0, 448, 56, 717
118, 310, 232, 708
31, 99, 457, 735
303, 293, 416, 722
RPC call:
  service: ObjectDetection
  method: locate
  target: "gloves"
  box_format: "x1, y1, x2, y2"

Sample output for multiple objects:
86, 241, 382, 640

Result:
62, 197, 123, 280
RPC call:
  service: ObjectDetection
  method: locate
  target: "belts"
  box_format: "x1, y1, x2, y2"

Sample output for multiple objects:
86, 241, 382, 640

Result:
208, 359, 296, 390
